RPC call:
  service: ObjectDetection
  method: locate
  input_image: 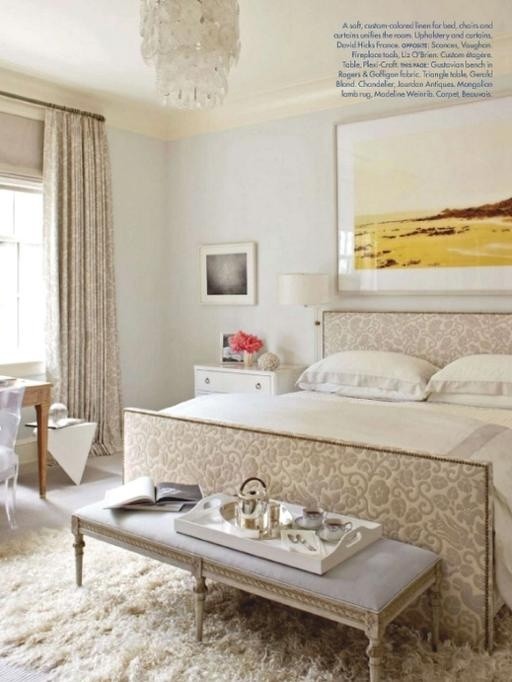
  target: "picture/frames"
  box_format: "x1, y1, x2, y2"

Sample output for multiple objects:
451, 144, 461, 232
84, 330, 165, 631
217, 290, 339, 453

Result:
197, 242, 257, 307
219, 331, 244, 365
333, 89, 512, 298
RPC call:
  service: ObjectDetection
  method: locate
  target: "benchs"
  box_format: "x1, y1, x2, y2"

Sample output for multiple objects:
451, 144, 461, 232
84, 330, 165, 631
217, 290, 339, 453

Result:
70, 499, 443, 682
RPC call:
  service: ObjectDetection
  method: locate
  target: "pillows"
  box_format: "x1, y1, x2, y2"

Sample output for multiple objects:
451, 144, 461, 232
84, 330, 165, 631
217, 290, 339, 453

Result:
294, 351, 439, 402
426, 353, 512, 410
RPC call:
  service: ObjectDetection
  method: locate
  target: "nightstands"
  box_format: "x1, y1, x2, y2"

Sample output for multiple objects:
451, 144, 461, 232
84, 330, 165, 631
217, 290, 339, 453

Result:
193, 363, 308, 397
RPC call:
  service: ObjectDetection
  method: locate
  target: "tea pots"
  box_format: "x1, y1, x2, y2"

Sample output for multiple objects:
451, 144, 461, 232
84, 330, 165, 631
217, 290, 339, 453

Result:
257, 500, 282, 538
232, 476, 270, 531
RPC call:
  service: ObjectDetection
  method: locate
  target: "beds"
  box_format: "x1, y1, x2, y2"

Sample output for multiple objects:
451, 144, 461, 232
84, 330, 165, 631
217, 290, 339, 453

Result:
120, 307, 512, 654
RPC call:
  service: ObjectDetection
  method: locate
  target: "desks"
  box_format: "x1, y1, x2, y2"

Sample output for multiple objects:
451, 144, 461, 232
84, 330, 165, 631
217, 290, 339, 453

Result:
0, 375, 54, 498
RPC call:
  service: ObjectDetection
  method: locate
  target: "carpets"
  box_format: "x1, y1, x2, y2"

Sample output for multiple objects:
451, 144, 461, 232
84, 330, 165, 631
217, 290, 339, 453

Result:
0, 509, 512, 681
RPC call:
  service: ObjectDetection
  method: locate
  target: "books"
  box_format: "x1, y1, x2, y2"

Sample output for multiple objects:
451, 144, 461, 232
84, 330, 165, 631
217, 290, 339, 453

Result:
102, 476, 202, 512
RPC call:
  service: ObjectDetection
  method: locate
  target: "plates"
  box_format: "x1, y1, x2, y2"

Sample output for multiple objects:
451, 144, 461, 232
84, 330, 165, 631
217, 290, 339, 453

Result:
293, 517, 328, 530
315, 524, 353, 543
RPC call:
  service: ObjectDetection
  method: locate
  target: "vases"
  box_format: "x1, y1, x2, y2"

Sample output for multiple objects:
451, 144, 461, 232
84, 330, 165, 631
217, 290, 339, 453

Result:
243, 351, 257, 368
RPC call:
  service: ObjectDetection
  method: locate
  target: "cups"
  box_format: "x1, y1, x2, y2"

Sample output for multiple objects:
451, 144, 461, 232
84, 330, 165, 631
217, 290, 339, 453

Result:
303, 506, 330, 529
322, 517, 354, 541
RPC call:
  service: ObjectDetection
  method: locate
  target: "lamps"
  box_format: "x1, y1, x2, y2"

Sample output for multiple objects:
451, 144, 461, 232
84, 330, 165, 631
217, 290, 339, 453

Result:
139, 0, 241, 113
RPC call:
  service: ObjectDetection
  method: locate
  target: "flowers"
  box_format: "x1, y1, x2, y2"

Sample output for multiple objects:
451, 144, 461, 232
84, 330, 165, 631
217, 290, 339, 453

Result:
228, 330, 263, 361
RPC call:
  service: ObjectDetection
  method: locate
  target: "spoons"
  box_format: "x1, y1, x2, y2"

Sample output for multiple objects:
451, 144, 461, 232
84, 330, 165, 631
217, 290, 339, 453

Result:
296, 533, 318, 552
288, 533, 314, 559
